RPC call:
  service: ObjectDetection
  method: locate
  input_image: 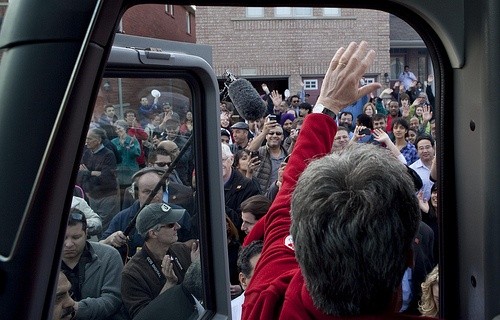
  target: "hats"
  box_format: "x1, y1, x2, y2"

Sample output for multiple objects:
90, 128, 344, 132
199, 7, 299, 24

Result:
136, 203, 186, 233
230, 122, 249, 130
166, 120, 180, 127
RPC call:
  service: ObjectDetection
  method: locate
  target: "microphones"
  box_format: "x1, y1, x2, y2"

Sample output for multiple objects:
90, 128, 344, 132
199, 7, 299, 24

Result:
229, 78, 268, 123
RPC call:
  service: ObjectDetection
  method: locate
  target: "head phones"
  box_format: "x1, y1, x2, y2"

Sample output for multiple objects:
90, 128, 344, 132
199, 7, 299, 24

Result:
128, 167, 169, 199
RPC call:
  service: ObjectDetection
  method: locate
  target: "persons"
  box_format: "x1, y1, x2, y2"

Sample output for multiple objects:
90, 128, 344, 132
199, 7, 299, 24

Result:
51, 41, 442, 320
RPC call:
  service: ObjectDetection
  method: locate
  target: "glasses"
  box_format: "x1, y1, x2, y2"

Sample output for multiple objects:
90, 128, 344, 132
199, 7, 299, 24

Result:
269, 131, 281, 135
71, 213, 86, 224
152, 162, 171, 167
167, 127, 177, 130
161, 223, 174, 228
221, 139, 229, 143
292, 100, 299, 103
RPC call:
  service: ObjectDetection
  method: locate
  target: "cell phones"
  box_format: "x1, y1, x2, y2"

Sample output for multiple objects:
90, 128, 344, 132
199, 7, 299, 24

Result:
359, 128, 371, 135
252, 151, 260, 166
269, 115, 277, 127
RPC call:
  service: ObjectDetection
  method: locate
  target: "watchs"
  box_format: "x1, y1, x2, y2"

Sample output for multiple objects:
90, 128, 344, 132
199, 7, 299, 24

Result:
312, 103, 337, 120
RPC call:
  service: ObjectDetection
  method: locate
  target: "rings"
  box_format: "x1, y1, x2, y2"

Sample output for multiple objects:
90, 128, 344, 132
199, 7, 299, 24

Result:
252, 163, 255, 166
